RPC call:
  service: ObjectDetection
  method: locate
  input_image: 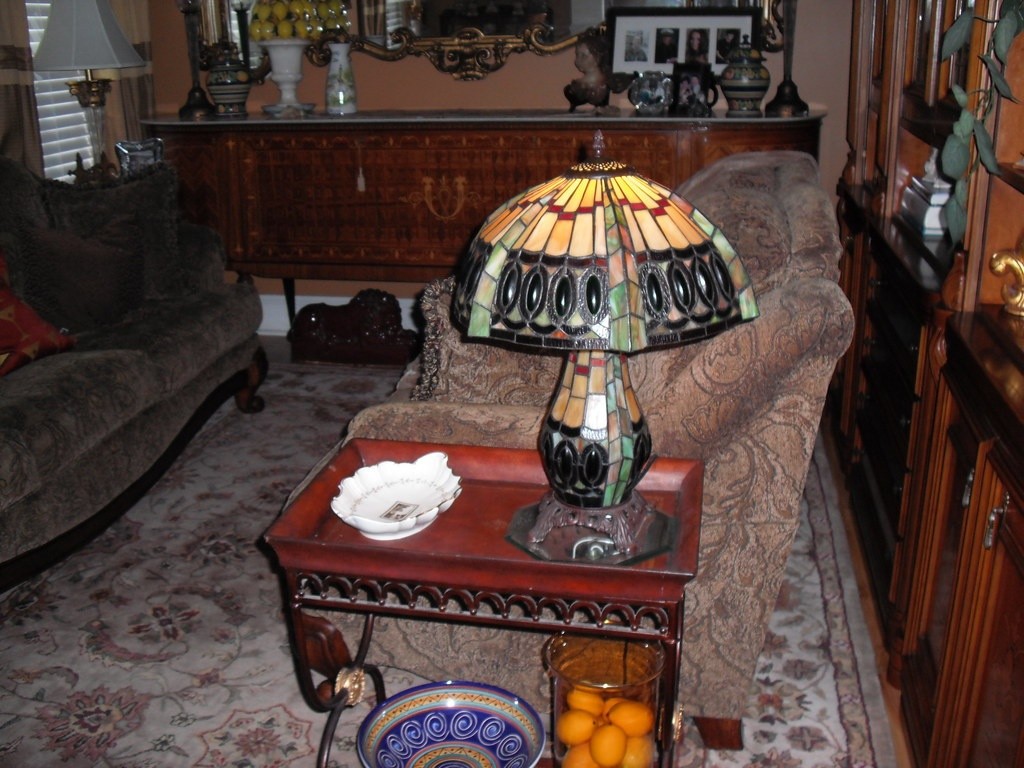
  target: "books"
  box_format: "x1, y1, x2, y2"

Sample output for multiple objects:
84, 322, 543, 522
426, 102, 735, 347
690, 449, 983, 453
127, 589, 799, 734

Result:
901, 176, 955, 239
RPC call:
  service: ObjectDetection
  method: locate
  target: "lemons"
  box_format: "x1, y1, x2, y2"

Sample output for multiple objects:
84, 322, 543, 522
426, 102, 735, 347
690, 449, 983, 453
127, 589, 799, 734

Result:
249, 0, 352, 42
557, 683, 657, 768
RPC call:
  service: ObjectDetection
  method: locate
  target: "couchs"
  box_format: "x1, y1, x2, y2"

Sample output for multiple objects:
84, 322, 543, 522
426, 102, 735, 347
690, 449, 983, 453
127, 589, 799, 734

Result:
0, 154, 268, 594
281, 146, 856, 752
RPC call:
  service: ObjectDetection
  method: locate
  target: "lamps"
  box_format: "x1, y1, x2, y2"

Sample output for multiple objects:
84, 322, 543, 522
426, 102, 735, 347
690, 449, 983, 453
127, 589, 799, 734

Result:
450, 130, 761, 565
177, 0, 215, 118
31, 0, 147, 174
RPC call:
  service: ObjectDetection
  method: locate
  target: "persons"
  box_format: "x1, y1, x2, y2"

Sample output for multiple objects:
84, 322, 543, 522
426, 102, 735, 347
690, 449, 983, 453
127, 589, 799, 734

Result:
924, 148, 938, 181
625, 34, 648, 61
716, 29, 739, 64
655, 28, 678, 63
686, 27, 709, 64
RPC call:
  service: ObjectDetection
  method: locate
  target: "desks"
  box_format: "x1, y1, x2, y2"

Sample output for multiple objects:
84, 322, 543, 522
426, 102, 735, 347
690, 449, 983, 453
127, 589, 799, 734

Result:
263, 438, 704, 768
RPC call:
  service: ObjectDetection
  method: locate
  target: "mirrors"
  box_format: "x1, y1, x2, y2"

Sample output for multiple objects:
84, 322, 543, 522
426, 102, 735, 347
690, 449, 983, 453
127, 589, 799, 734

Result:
188, 0, 786, 82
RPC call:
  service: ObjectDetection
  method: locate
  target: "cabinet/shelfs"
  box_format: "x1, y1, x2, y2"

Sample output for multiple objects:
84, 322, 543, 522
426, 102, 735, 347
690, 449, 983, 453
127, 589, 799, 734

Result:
142, 108, 829, 322
821, 0, 1024, 768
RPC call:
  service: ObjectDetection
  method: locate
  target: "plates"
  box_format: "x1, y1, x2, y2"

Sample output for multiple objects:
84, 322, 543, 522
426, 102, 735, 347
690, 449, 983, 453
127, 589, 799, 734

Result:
331, 451, 463, 540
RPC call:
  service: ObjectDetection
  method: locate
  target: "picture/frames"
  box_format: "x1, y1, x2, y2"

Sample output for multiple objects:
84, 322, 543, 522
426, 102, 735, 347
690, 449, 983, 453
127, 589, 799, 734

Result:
671, 62, 710, 112
605, 6, 763, 83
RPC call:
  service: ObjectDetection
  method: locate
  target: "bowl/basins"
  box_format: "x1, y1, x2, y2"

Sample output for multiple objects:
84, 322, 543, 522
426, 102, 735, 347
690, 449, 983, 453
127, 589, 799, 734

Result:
357, 681, 546, 768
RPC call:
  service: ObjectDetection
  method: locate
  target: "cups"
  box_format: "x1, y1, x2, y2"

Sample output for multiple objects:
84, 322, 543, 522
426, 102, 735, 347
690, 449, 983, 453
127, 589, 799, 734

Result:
544, 632, 667, 768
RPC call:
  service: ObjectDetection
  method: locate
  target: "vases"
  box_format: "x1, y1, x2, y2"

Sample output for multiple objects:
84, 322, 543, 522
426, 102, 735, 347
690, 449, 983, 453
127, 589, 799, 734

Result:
324, 42, 357, 115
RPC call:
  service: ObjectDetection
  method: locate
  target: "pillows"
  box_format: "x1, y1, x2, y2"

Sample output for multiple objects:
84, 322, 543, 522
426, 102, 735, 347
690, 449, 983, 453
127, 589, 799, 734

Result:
413, 276, 566, 406
17, 213, 147, 336
51, 163, 198, 302
1, 259, 80, 379
